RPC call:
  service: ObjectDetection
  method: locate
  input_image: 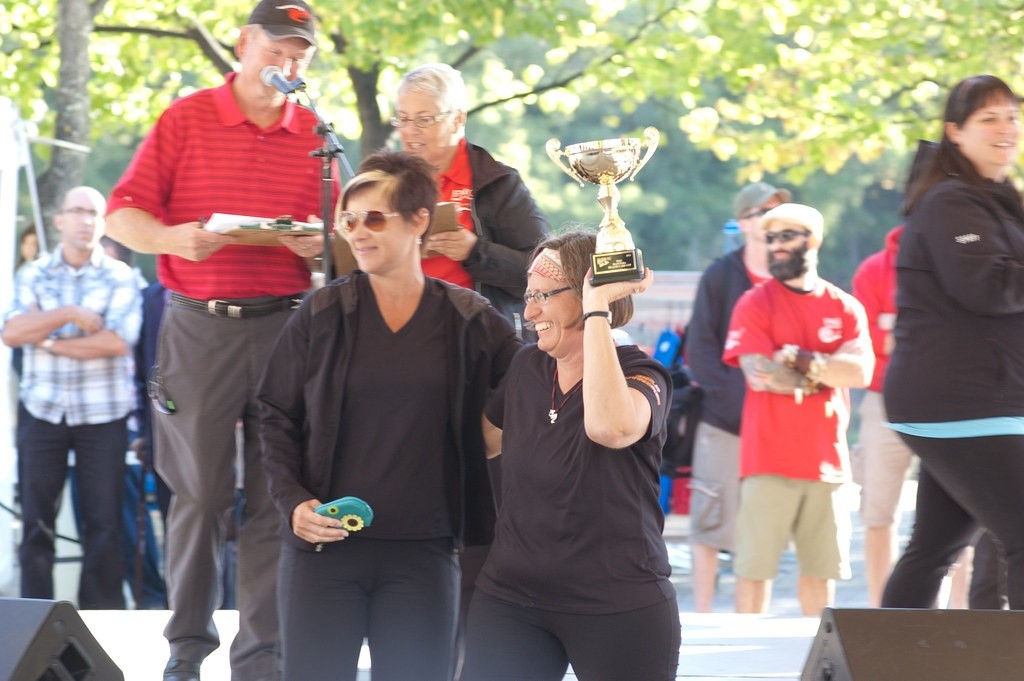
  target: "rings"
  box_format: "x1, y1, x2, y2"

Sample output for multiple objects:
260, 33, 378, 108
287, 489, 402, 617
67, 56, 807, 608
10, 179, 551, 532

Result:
634, 287, 640, 295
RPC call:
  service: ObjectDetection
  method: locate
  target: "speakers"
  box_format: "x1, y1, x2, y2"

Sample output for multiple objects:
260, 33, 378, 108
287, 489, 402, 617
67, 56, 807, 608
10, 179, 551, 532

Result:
799, 608, 1024, 681
0, 596, 124, 681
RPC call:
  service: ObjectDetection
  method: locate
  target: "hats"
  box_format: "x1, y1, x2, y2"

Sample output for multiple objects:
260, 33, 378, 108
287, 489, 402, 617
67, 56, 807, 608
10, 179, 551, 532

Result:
245, 0, 317, 47
760, 203, 824, 245
733, 183, 793, 220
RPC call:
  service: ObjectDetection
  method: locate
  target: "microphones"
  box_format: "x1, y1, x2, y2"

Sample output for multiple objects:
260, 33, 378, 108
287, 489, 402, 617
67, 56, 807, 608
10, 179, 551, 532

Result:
259, 65, 295, 97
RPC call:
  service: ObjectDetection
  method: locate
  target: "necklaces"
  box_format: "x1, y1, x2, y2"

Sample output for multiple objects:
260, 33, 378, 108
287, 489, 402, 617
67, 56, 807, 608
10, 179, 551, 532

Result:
549, 369, 582, 424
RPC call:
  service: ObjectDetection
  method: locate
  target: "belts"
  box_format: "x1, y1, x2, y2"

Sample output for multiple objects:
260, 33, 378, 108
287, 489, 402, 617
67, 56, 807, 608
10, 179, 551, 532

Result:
169, 293, 304, 319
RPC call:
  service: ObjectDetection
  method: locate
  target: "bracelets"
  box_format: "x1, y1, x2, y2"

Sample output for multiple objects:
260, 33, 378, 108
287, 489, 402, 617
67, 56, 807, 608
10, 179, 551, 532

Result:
582, 311, 614, 325
783, 344, 830, 384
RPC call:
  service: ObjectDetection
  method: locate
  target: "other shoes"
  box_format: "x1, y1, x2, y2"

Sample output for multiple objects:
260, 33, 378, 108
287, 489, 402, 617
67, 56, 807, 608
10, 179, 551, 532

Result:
163, 656, 202, 681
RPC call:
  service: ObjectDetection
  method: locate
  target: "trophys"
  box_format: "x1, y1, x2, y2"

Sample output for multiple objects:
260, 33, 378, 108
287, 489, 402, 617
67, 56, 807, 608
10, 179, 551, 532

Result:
545, 124, 660, 287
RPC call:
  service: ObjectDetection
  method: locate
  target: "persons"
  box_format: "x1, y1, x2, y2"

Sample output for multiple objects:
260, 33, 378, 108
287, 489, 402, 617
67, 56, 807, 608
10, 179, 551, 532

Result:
721, 202, 876, 617
68, 236, 168, 610
103, 0, 340, 681
880, 74, 1024, 612
398, 63, 550, 342
1, 187, 143, 610
853, 225, 976, 609
257, 143, 534, 681
683, 178, 792, 612
460, 217, 682, 680
11, 225, 41, 380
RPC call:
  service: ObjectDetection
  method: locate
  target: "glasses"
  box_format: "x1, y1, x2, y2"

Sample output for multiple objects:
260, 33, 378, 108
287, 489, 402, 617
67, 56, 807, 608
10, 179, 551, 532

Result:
523, 286, 574, 305
390, 110, 460, 129
58, 207, 103, 219
146, 366, 176, 416
765, 229, 808, 244
740, 208, 771, 220
334, 209, 402, 235
